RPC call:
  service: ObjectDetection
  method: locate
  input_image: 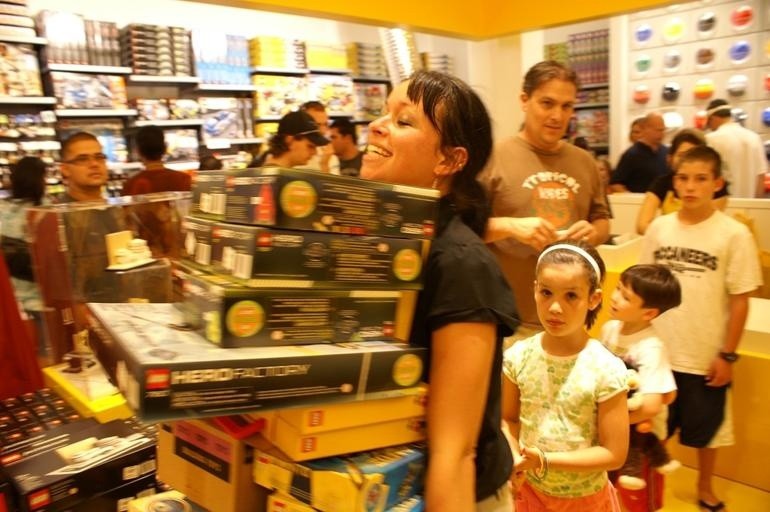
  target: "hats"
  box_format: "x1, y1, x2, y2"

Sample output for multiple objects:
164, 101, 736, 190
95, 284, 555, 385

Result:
702, 104, 730, 128
278, 111, 331, 146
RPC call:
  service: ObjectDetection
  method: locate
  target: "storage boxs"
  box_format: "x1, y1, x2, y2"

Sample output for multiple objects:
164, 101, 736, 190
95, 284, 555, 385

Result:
252, 447, 424, 512
166, 259, 421, 349
0, 419, 156, 511
266, 492, 421, 512
180, 215, 433, 291
153, 421, 267, 512
192, 168, 442, 240
85, 301, 431, 424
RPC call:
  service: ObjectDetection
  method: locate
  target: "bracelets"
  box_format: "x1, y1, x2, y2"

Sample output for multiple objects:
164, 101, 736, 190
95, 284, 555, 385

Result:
525, 445, 548, 483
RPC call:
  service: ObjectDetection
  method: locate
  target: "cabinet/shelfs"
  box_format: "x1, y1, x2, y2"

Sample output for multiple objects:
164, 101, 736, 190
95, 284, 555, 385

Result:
41, 61, 137, 197
568, 83, 608, 151
0, 38, 62, 197
312, 69, 354, 117
250, 67, 310, 155
128, 72, 203, 176
194, 83, 261, 169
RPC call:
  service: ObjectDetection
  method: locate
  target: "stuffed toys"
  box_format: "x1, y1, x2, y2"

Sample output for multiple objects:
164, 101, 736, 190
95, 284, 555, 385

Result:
618, 362, 682, 491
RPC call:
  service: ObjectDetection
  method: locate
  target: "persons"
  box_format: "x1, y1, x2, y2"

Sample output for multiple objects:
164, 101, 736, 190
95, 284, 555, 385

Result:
608, 114, 671, 195
246, 102, 364, 178
597, 159, 629, 194
599, 263, 678, 512
359, 71, 519, 511
474, 61, 615, 349
119, 125, 191, 196
56, 132, 109, 203
635, 129, 731, 235
705, 98, 770, 198
500, 239, 630, 512
10, 156, 46, 206
637, 147, 765, 512
629, 117, 645, 144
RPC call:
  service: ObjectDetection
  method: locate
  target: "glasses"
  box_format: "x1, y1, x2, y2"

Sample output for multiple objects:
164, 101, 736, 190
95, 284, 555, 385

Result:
62, 154, 107, 165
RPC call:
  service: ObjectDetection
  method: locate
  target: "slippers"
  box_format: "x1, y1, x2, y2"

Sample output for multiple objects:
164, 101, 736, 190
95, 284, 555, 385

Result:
698, 500, 724, 510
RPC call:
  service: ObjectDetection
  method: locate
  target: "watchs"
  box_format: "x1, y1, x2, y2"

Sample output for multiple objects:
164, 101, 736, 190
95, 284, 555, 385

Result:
719, 351, 739, 362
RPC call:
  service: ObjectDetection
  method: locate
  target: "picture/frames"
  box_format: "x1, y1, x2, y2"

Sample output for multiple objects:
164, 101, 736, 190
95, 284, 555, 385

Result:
350, 78, 391, 149
